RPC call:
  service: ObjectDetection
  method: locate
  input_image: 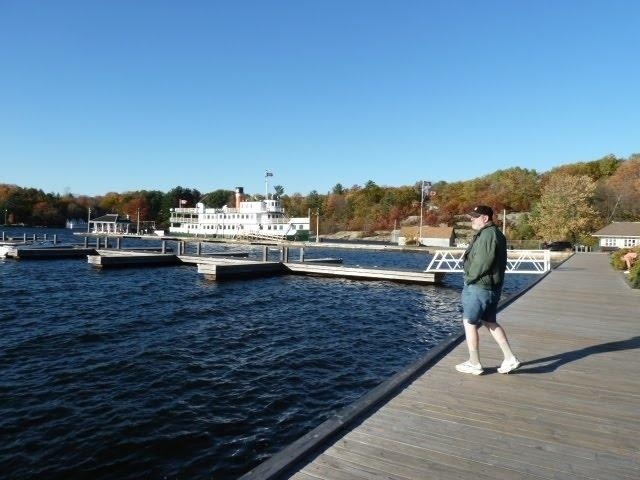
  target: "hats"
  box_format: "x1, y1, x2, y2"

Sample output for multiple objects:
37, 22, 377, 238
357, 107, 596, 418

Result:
468, 205, 493, 218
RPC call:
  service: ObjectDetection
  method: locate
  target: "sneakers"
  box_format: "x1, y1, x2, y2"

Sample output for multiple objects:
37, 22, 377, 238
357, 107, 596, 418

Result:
456, 361, 485, 375
497, 357, 521, 373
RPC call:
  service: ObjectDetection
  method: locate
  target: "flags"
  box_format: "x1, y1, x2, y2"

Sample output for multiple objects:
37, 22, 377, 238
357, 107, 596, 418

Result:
267, 172, 274, 176
181, 200, 188, 204
423, 181, 432, 187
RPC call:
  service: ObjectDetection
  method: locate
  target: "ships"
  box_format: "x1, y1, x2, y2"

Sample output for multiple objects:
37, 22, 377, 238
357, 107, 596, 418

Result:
169, 169, 311, 240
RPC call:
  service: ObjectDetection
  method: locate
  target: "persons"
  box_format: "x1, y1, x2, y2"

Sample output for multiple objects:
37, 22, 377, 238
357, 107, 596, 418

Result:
620, 252, 638, 268
455, 205, 520, 376
